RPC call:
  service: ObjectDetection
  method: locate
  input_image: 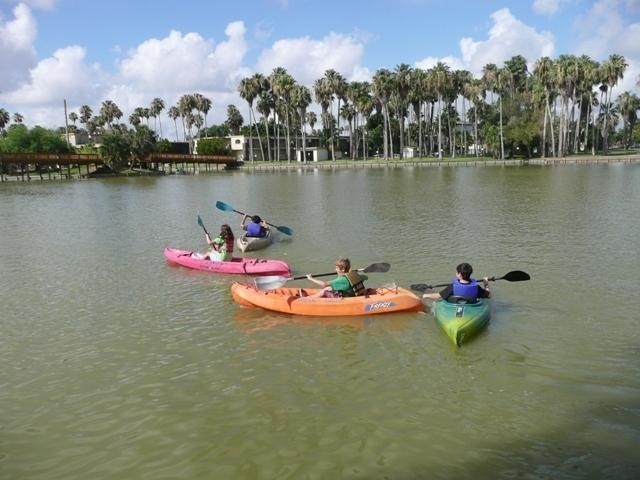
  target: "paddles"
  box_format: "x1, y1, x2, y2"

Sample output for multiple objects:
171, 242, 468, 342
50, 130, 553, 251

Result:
409, 271, 530, 292
216, 201, 293, 235
197, 213, 216, 252
254, 262, 390, 290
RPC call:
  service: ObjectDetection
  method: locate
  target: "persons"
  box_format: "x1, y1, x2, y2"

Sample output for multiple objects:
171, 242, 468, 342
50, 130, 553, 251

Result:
241, 214, 269, 237
191, 225, 233, 262
422, 263, 489, 304
300, 257, 369, 297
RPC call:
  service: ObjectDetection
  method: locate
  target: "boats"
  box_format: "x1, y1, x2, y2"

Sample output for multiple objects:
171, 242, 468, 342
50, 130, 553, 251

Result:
236, 220, 271, 253
231, 280, 423, 316
164, 246, 293, 277
431, 296, 490, 347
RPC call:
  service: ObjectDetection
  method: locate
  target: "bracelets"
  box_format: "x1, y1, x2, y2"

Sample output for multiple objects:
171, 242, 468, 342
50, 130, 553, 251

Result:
484, 284, 487, 287
